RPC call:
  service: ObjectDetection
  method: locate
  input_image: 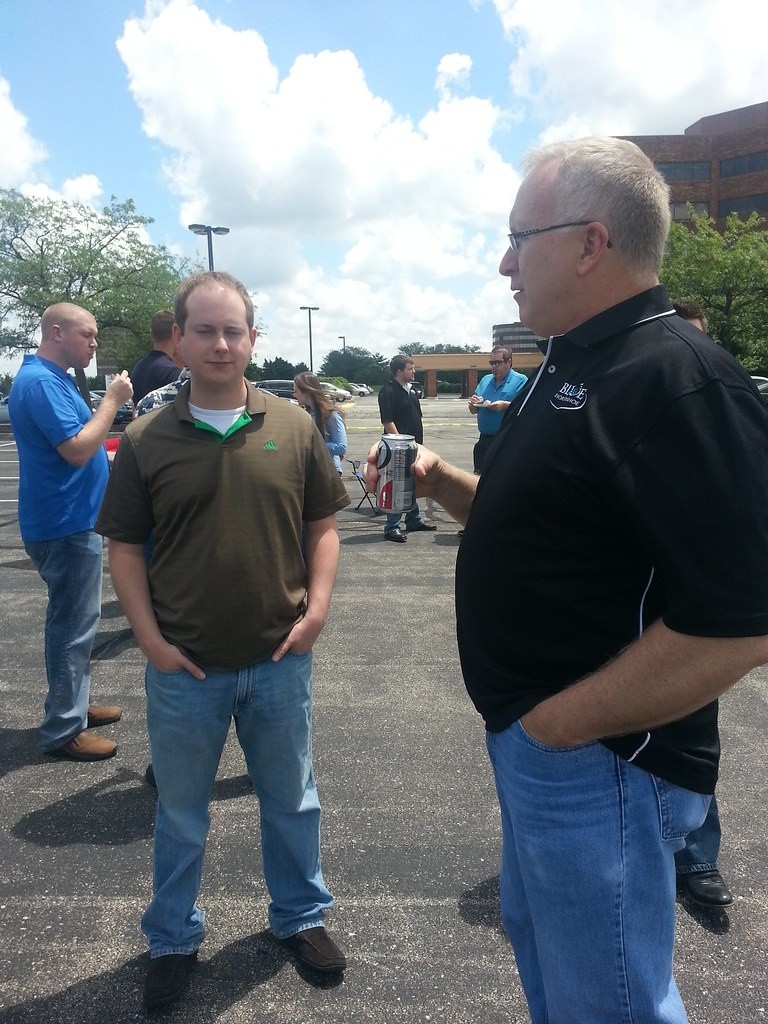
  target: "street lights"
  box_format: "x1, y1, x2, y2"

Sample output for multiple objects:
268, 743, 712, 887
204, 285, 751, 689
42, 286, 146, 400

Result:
188, 223, 230, 273
300, 304, 320, 380
338, 336, 346, 352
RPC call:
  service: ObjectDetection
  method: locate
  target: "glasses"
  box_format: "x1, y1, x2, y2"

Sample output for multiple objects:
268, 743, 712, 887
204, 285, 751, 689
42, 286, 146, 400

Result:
507, 221, 611, 254
489, 359, 504, 366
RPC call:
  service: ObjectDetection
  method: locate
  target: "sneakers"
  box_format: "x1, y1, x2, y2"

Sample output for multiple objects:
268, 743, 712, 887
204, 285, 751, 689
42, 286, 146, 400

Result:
285, 927, 347, 971
146, 949, 199, 1006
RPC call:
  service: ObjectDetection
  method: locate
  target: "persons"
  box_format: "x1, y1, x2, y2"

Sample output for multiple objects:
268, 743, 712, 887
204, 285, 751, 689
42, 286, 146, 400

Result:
376, 354, 437, 542
294, 372, 347, 589
359, 136, 768, 1024
93, 274, 351, 1011
660, 296, 734, 906
467, 347, 528, 473
8, 302, 133, 759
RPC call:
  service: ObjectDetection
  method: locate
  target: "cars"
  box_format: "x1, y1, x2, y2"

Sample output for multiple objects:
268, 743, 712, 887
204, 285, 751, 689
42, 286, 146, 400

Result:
87, 391, 132, 424
319, 382, 352, 402
348, 383, 373, 397
409, 382, 424, 397
255, 379, 297, 399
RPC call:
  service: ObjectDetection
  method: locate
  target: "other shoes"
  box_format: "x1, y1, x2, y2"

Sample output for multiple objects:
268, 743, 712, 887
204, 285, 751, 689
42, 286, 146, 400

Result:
457, 529, 464, 535
146, 764, 157, 788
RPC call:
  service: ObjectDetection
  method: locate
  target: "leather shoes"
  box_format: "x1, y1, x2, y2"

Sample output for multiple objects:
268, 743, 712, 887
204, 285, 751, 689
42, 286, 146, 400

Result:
682, 869, 733, 907
385, 531, 407, 541
407, 523, 436, 531
46, 730, 118, 758
88, 704, 122, 725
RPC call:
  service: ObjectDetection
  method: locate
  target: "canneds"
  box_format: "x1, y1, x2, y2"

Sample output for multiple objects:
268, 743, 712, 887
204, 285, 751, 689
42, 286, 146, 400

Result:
375, 433, 419, 514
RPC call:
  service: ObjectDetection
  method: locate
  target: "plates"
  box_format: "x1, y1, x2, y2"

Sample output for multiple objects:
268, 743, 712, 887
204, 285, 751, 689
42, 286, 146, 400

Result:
473, 404, 488, 407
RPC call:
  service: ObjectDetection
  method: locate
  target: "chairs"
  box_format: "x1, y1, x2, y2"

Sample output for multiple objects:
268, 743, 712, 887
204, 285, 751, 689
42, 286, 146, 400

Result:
347, 459, 380, 514
